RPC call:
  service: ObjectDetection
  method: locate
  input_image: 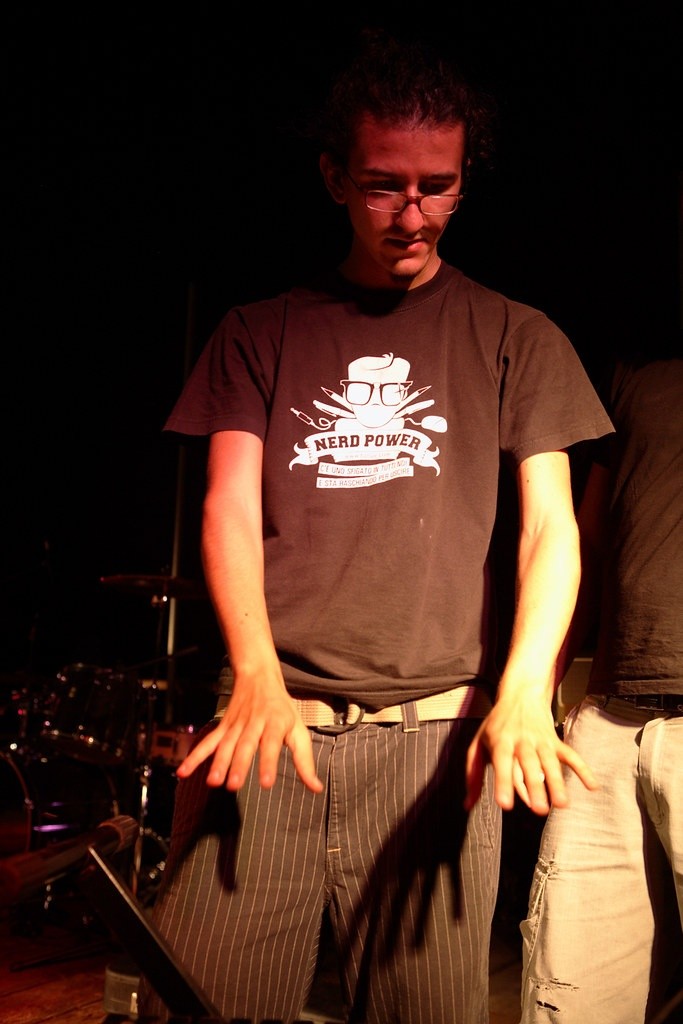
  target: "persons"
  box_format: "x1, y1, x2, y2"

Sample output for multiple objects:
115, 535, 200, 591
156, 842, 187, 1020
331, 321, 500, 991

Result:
515, 354, 683, 1024
155, 52, 617, 1024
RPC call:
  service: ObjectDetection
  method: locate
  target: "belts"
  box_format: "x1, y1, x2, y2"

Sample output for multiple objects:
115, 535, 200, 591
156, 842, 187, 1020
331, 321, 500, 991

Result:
606, 693, 683, 713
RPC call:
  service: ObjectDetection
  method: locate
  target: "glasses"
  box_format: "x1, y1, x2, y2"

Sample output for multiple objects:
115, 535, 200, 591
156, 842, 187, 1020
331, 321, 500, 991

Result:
344, 169, 464, 216
